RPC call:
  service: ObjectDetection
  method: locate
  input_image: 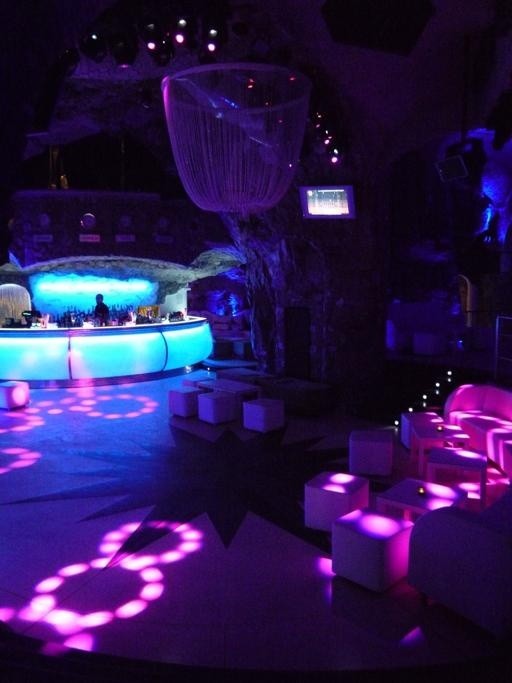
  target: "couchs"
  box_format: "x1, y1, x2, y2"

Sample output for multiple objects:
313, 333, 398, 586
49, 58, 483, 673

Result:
407, 383, 512, 639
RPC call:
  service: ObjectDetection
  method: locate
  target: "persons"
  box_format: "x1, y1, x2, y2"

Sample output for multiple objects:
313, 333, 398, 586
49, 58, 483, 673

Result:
93, 294, 110, 325
384, 235, 478, 422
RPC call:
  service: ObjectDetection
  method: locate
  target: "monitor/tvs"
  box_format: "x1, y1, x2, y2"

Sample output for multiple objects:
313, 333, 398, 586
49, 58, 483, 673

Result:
298, 185, 358, 221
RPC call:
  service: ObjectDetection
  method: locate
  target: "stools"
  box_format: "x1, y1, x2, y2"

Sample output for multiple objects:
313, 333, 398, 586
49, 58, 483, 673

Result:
401, 412, 445, 449
0, 380, 30, 411
169, 386, 285, 434
304, 430, 468, 595
183, 376, 214, 386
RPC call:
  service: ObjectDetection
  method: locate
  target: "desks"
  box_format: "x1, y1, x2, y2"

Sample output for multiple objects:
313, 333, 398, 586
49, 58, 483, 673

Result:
216, 368, 260, 384
196, 378, 262, 401
410, 425, 488, 510
